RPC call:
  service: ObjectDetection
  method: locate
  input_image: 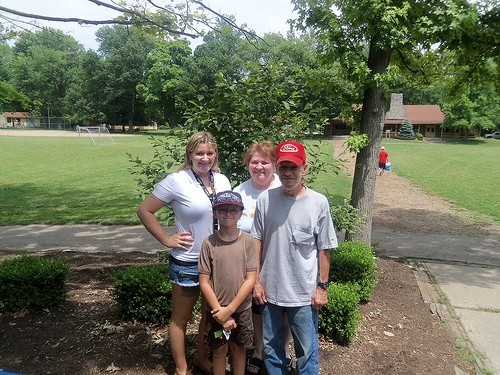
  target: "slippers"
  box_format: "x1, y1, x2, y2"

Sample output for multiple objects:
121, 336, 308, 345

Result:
192, 359, 214, 375
286, 354, 292, 373
246, 350, 263, 375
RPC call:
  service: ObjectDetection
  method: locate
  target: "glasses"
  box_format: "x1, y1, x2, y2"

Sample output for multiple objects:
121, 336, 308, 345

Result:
216, 206, 240, 215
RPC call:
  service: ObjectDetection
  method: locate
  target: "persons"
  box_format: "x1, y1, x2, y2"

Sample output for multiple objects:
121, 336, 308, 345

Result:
378, 146, 389, 175
231, 140, 292, 375
198, 190, 260, 375
249, 140, 338, 375
136, 132, 232, 375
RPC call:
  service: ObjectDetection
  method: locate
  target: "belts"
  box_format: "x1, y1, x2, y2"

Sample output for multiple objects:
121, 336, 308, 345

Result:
169, 254, 198, 266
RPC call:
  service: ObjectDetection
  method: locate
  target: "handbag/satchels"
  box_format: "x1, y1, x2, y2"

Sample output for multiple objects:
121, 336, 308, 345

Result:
385, 162, 391, 171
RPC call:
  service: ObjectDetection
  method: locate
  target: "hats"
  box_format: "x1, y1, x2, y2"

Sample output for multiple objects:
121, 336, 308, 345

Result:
212, 190, 244, 210
381, 146, 384, 149
276, 140, 306, 167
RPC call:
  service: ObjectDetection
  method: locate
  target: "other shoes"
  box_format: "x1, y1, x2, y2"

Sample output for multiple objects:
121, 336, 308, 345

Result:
380, 172, 382, 175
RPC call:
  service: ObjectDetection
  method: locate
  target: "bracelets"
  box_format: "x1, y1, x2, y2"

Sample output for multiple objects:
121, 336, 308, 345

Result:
318, 282, 329, 290
